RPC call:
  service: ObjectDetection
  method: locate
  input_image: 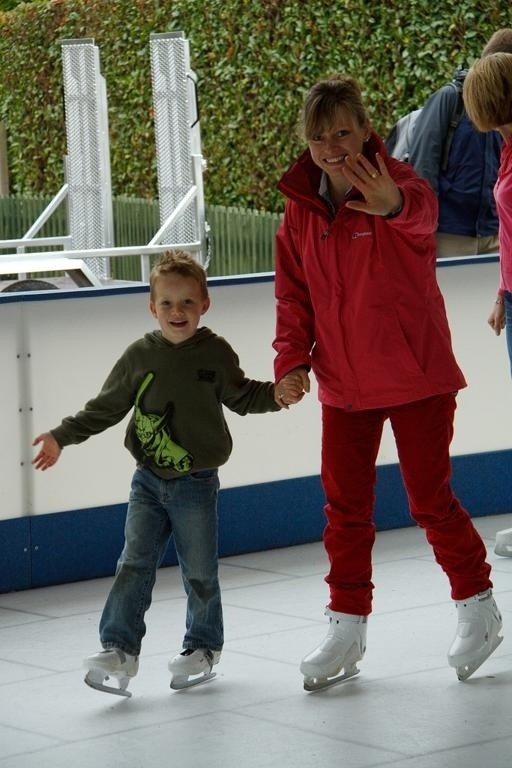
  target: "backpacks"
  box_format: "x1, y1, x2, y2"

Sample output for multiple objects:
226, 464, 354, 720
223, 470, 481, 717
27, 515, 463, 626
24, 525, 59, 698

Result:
386, 79, 464, 163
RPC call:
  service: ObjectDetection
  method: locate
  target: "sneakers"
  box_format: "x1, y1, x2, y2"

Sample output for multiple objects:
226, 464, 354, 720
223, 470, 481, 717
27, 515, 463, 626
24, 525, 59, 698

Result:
167, 644, 221, 676
299, 606, 368, 677
86, 647, 140, 680
447, 586, 503, 667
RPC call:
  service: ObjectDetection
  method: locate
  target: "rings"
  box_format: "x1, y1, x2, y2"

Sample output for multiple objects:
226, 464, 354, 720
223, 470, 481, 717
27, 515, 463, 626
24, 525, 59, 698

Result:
371, 169, 379, 179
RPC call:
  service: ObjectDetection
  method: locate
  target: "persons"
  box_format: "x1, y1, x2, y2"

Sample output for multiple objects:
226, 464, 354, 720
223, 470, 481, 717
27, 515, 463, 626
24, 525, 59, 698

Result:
31, 244, 308, 676
266, 76, 504, 680
404, 25, 511, 257
461, 48, 512, 550
